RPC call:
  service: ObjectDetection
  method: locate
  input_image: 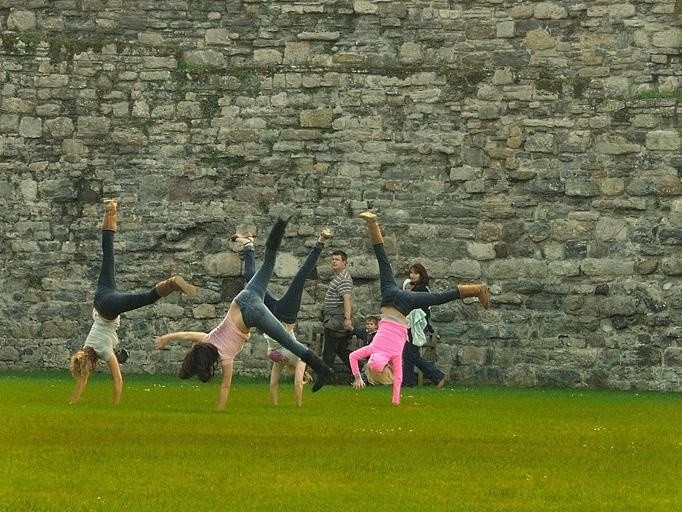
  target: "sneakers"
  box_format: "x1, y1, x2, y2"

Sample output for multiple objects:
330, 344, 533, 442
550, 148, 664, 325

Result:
437, 375, 448, 388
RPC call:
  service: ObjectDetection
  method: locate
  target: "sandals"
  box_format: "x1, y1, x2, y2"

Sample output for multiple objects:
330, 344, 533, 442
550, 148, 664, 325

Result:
231, 208, 331, 250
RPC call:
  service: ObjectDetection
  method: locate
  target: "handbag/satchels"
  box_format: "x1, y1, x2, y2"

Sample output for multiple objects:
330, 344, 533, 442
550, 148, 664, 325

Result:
423, 330, 438, 346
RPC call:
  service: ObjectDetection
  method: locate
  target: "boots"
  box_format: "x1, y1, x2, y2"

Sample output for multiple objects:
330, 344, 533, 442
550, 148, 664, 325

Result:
359, 212, 383, 245
301, 348, 334, 393
102, 198, 117, 232
457, 285, 488, 309
156, 275, 196, 298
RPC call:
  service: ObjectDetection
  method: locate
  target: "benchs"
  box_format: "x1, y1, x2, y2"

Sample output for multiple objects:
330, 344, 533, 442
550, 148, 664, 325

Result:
313, 328, 438, 387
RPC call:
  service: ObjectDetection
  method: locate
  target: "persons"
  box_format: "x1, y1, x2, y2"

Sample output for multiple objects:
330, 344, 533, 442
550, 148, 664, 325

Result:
226, 226, 332, 408
67, 198, 197, 405
323, 251, 449, 388
348, 211, 490, 408
154, 207, 335, 411
